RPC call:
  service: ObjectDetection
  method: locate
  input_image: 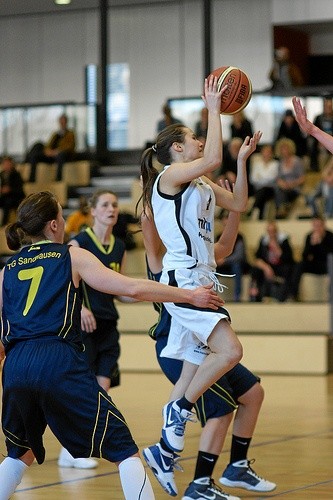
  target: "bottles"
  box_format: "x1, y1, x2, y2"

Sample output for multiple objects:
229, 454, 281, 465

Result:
250, 280, 258, 301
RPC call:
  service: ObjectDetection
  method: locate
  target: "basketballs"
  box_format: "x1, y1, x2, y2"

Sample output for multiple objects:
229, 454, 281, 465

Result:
206, 67, 252, 116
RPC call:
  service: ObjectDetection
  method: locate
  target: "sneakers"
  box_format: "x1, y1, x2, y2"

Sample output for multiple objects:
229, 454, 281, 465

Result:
142, 442, 184, 497
57, 447, 98, 469
181, 479, 240, 500
219, 458, 276, 492
161, 398, 197, 452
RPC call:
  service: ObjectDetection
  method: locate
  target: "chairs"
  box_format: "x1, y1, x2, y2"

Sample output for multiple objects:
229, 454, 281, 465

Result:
0, 159, 91, 255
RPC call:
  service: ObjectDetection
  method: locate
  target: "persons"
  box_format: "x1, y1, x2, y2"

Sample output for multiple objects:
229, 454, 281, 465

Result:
0, 191, 225, 500
154, 92, 333, 304
57, 189, 140, 468
112, 212, 140, 249
135, 74, 276, 500
268, 47, 302, 89
0, 158, 26, 227
28, 115, 75, 183
63, 196, 95, 243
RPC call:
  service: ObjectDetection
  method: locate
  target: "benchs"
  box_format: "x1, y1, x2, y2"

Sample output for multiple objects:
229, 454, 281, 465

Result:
140, 149, 333, 304
